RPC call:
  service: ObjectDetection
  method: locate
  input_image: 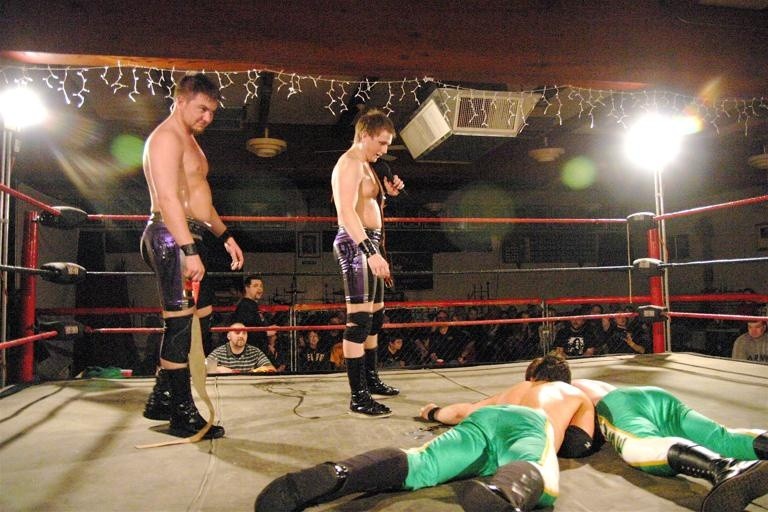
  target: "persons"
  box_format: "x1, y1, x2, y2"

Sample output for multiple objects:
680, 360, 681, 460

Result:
732, 288, 768, 365
328, 107, 407, 420
137, 73, 247, 442
566, 378, 768, 511
204, 276, 346, 376
251, 352, 596, 512
379, 307, 650, 368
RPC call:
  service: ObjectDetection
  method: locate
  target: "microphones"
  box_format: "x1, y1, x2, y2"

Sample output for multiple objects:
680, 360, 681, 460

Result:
378, 158, 412, 199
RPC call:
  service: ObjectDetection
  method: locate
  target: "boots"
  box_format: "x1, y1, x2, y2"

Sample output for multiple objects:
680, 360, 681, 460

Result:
363, 345, 399, 395
143, 368, 170, 420
253, 448, 407, 512
162, 368, 224, 440
667, 441, 768, 512
344, 354, 393, 418
457, 462, 543, 511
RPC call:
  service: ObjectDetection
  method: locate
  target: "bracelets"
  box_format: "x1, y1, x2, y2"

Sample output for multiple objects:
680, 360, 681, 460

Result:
177, 241, 201, 255
213, 224, 233, 247
355, 237, 377, 258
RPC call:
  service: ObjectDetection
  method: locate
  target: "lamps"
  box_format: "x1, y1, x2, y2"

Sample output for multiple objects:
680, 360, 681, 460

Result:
528, 135, 566, 163
243, 127, 286, 158
747, 143, 768, 172
